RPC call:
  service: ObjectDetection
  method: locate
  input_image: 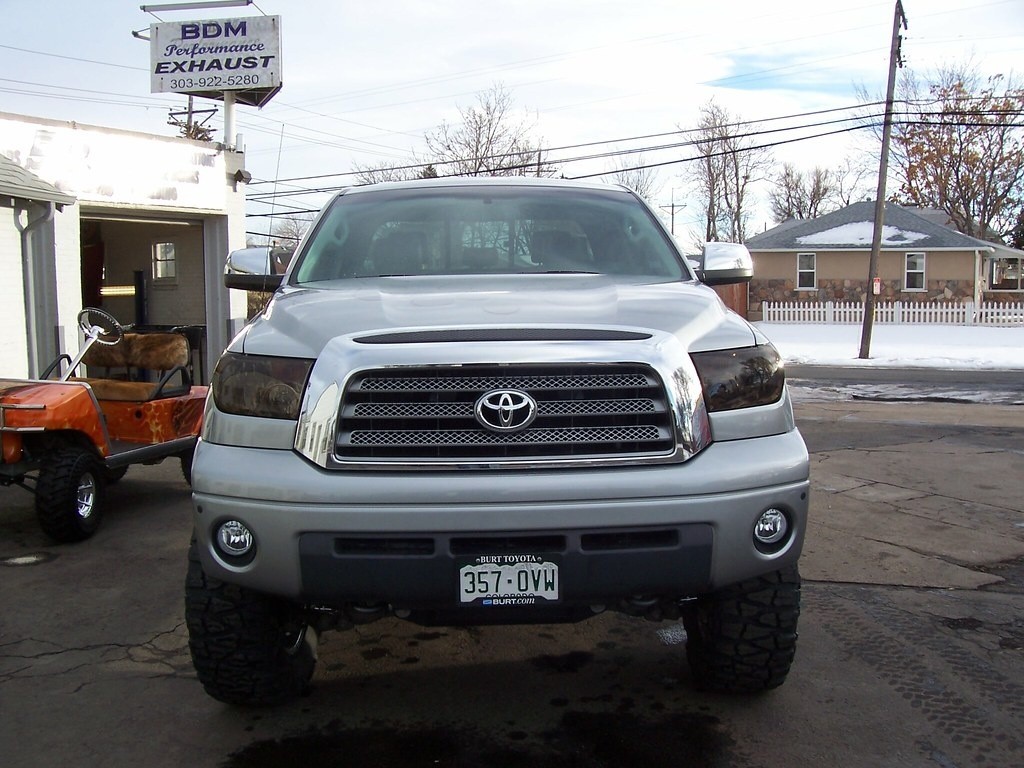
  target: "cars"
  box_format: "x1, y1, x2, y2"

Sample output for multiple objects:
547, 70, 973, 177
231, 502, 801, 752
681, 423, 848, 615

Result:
0, 304, 209, 546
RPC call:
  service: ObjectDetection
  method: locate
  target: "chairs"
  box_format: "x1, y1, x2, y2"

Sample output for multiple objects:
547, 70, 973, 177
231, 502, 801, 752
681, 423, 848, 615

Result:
233, 169, 252, 186
458, 247, 500, 272
370, 231, 432, 274
530, 230, 589, 269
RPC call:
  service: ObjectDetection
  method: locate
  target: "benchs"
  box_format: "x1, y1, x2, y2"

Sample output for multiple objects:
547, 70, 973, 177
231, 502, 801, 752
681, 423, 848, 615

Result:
42, 330, 193, 403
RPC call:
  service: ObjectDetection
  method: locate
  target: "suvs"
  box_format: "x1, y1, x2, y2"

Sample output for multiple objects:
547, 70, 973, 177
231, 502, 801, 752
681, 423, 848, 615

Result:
182, 174, 814, 710
